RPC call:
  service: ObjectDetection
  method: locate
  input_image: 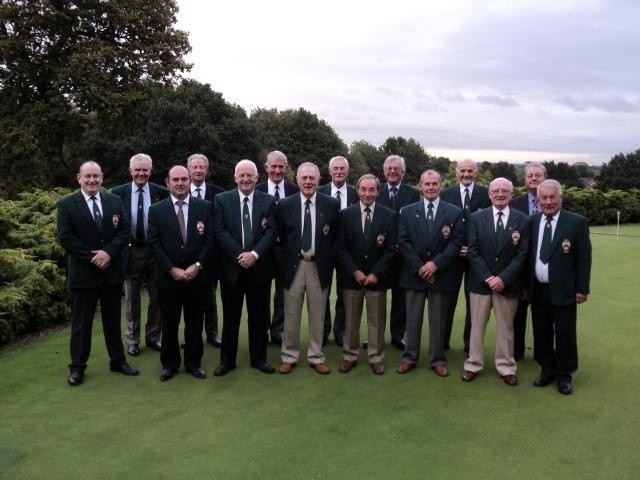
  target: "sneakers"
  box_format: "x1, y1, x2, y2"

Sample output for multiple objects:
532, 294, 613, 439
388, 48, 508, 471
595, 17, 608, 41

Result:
321, 337, 368, 347
180, 337, 222, 348
271, 335, 282, 346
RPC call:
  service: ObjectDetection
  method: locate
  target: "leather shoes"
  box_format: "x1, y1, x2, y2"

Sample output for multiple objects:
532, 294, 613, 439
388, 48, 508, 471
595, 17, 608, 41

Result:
128, 343, 140, 356
68, 364, 87, 386
109, 359, 139, 376
146, 341, 161, 352
339, 359, 357, 373
159, 366, 179, 381
213, 363, 238, 376
462, 370, 479, 382
430, 365, 449, 377
557, 378, 572, 394
185, 366, 207, 379
370, 362, 385, 375
250, 361, 275, 374
500, 375, 519, 386
309, 363, 331, 375
534, 375, 556, 387
278, 361, 296, 374
396, 361, 417, 374
392, 339, 407, 351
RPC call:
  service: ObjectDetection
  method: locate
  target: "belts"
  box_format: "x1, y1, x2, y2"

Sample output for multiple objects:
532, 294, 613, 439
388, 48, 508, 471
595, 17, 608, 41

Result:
298, 255, 318, 263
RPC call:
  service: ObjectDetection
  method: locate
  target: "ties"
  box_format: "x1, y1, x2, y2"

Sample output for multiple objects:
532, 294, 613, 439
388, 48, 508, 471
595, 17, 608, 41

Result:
135, 188, 146, 241
496, 211, 505, 251
390, 186, 400, 207
300, 198, 313, 253
274, 185, 280, 202
426, 202, 435, 226
539, 216, 555, 265
464, 188, 472, 217
363, 206, 373, 243
242, 196, 253, 251
90, 195, 104, 233
335, 190, 342, 212
175, 201, 187, 240
195, 188, 202, 199
531, 198, 540, 216
